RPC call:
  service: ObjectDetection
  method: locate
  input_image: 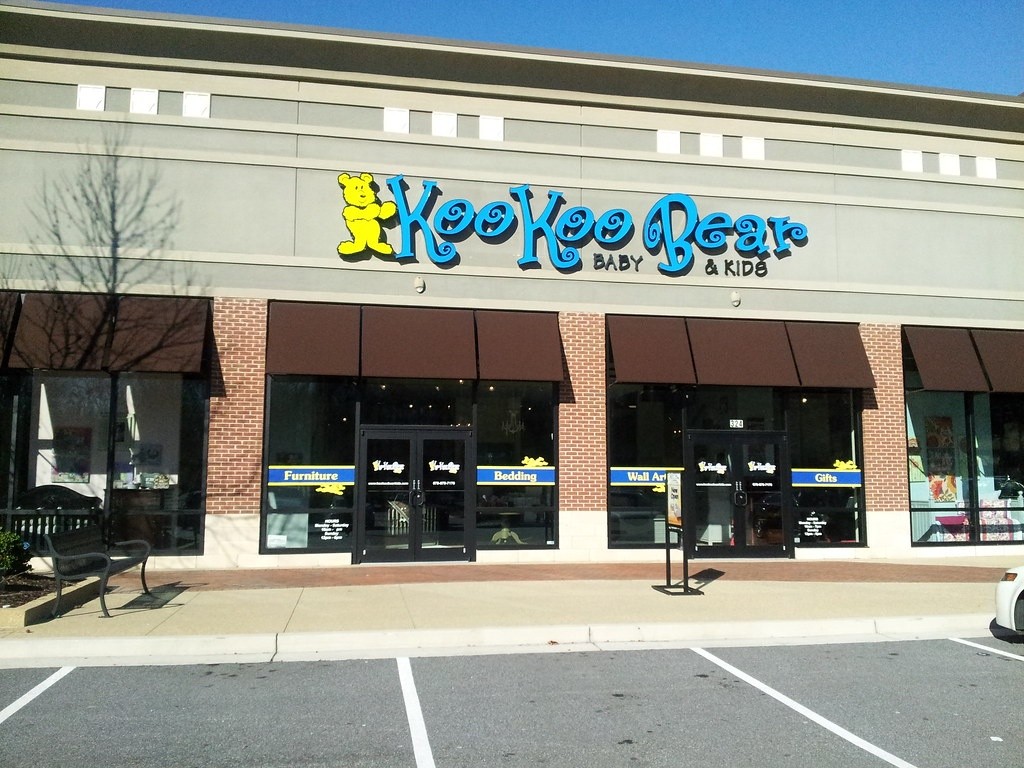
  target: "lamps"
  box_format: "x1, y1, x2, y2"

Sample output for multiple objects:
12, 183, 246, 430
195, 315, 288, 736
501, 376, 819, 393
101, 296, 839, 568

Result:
730, 291, 740, 306
414, 276, 424, 293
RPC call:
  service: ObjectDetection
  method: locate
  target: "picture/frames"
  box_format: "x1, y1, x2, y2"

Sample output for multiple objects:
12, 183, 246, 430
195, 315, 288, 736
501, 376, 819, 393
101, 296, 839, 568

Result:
97, 412, 135, 452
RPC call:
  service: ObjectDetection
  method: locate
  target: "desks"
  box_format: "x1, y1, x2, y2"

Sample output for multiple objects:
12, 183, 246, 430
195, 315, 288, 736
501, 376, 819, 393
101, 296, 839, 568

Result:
475, 505, 554, 545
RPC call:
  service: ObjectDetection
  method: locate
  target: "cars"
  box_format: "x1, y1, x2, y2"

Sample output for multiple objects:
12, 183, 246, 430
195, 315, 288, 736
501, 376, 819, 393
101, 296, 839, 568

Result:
995, 566, 1024, 635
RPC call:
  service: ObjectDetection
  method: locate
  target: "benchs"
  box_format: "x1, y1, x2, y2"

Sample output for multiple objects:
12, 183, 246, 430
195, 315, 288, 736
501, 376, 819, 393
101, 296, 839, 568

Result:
43, 524, 160, 618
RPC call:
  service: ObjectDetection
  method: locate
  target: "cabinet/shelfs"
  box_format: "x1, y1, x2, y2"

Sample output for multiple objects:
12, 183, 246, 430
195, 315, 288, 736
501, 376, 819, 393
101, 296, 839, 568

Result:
103, 488, 176, 549
